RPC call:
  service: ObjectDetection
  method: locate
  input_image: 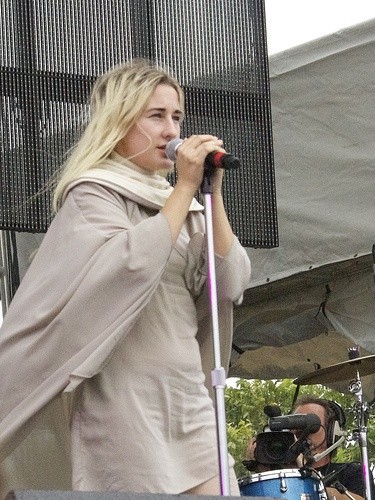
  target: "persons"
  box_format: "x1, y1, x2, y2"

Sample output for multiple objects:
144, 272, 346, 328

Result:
245, 397, 375, 500
1, 59, 253, 497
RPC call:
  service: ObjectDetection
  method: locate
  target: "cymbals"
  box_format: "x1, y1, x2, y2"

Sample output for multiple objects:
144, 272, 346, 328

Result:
293, 355, 375, 385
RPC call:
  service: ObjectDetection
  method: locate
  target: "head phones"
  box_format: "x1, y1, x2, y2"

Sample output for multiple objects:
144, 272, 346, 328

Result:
322, 399, 347, 452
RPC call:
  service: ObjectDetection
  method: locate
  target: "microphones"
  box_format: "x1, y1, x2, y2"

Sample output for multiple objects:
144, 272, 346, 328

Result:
321, 464, 350, 487
312, 436, 345, 462
263, 405, 282, 416
164, 139, 241, 170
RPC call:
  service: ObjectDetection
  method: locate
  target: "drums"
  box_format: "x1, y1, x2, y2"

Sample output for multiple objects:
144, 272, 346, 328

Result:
237, 468, 327, 500
